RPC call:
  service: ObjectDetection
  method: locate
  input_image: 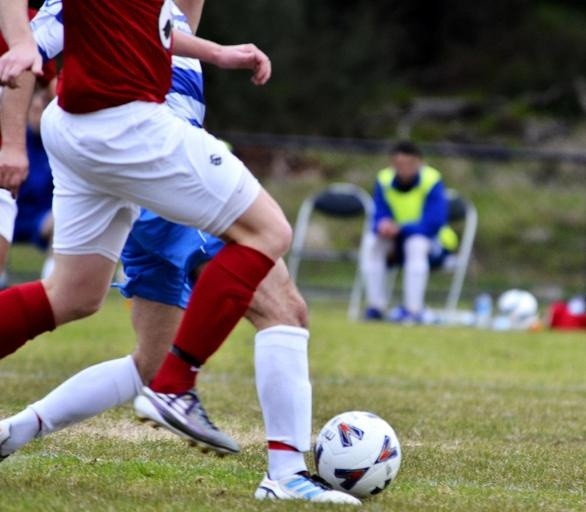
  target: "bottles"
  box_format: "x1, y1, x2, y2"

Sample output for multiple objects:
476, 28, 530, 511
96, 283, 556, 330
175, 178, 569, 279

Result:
473, 292, 495, 327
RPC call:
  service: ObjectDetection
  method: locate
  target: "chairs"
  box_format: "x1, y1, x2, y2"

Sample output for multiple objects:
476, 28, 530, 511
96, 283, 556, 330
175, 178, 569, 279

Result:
387, 189, 478, 312
289, 184, 373, 318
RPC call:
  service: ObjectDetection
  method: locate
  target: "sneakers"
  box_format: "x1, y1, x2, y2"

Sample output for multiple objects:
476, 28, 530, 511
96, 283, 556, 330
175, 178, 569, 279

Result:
251, 471, 364, 509
132, 386, 238, 461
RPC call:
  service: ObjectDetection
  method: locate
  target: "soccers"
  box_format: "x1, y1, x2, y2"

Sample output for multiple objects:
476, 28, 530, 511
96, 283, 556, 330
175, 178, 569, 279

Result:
314, 410, 402, 498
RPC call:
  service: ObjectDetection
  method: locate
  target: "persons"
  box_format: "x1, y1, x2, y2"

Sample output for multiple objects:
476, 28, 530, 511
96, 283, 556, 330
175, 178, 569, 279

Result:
356, 137, 461, 329
0, 0, 361, 504
